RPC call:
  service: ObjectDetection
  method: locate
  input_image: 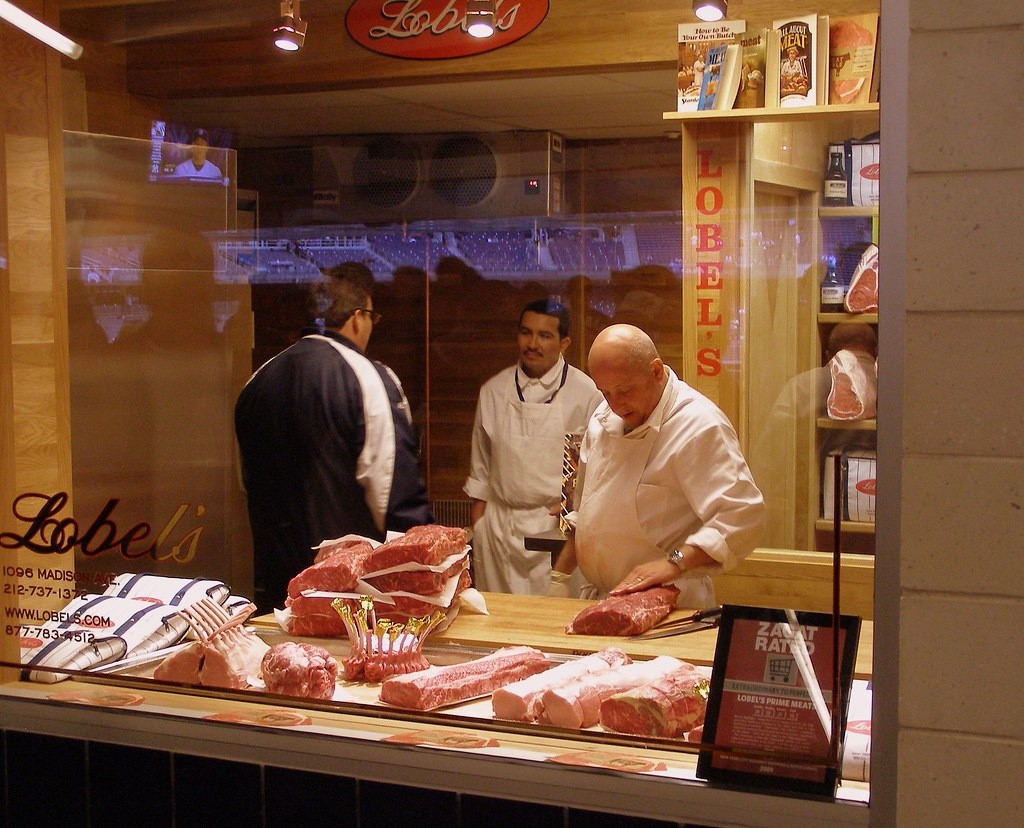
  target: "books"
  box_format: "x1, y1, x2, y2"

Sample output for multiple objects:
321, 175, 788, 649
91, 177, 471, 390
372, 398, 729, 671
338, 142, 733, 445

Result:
676, 19, 747, 112
828, 12, 881, 105
773, 14, 829, 108
698, 44, 743, 110
732, 28, 781, 109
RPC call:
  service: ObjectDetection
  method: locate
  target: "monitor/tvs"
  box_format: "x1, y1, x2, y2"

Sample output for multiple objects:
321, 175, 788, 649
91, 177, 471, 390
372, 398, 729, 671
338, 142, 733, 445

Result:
147, 121, 232, 188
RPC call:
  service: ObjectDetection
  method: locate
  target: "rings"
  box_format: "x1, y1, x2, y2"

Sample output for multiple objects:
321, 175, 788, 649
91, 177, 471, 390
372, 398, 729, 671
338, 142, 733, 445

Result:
636, 577, 641, 582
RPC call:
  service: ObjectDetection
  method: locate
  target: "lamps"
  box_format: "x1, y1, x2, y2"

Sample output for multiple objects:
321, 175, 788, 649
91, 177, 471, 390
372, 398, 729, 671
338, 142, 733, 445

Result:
467, 0, 495, 38
269, 0, 308, 51
692, 0, 728, 22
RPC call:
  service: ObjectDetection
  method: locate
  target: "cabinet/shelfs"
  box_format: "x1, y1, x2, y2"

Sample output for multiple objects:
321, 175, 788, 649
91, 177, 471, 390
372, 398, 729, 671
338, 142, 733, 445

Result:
814, 204, 880, 556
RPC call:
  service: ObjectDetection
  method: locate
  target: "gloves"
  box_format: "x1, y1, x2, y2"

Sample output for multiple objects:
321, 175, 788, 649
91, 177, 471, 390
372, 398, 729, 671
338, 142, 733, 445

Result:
546, 571, 572, 599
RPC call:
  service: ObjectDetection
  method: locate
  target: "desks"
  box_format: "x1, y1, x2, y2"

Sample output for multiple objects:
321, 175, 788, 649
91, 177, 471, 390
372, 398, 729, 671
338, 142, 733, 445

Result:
2, 594, 873, 828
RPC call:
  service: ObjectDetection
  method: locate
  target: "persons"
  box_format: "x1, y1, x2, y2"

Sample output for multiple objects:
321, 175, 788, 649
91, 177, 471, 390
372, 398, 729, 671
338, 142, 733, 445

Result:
694, 54, 706, 84
782, 45, 802, 80
754, 325, 878, 476
462, 298, 605, 599
678, 64, 687, 77
174, 130, 222, 176
87, 264, 112, 283
545, 322, 765, 610
234, 267, 437, 618
709, 62, 721, 84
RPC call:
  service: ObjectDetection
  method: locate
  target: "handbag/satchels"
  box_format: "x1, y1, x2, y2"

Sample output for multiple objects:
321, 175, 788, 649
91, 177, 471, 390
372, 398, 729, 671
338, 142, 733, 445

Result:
839, 680, 872, 784
823, 442, 876, 523
186, 595, 259, 640
18, 625, 128, 685
43, 594, 191, 661
103, 572, 233, 631
827, 130, 880, 207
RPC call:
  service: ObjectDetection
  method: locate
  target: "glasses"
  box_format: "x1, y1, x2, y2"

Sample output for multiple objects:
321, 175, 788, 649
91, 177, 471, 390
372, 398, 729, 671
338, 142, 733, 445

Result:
353, 309, 381, 324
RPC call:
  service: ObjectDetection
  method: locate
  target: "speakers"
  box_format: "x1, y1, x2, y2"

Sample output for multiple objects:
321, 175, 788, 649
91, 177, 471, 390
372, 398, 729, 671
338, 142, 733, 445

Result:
312, 129, 566, 221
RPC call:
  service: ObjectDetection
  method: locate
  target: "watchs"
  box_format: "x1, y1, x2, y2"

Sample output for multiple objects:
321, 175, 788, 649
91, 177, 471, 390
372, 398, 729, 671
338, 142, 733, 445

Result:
666, 549, 686, 575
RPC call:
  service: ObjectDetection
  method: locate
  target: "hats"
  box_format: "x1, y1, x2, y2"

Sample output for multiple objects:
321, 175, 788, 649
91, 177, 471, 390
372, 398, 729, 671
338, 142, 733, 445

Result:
193, 128, 209, 143
